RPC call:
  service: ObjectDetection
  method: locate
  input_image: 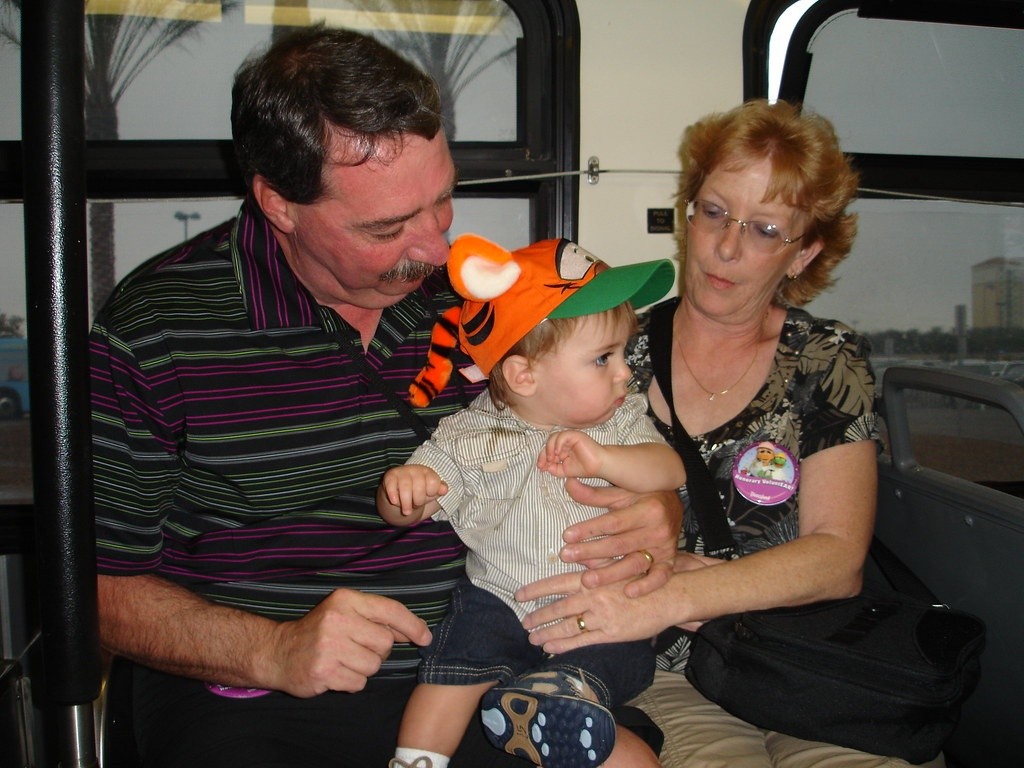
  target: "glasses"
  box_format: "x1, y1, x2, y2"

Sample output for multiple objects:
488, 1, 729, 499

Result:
684, 195, 812, 251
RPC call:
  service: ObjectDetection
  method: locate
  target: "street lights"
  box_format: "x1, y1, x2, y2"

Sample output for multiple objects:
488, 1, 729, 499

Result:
173, 210, 201, 242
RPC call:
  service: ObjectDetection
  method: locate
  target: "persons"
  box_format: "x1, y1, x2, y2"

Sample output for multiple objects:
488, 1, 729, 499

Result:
514, 101, 912, 768
88, 20, 683, 768
378, 238, 687, 768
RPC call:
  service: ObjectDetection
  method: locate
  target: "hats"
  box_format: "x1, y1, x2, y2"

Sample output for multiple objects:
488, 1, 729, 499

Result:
409, 234, 675, 410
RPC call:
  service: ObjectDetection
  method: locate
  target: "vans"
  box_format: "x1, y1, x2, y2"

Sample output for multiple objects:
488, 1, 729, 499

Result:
0, 338, 30, 420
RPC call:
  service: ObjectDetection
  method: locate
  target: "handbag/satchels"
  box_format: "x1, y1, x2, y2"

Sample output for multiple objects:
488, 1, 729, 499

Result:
685, 594, 987, 763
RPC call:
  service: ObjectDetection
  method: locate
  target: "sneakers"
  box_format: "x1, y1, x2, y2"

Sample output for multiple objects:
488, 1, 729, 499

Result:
481, 670, 616, 768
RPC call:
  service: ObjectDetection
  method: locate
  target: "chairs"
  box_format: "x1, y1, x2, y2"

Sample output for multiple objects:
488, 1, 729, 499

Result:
862, 364, 1023, 653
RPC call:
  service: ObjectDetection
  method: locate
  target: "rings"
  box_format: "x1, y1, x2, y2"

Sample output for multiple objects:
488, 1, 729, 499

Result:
576, 614, 589, 633
640, 549, 654, 564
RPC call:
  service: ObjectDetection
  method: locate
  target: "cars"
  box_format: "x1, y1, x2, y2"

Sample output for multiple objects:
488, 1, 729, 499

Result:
912, 356, 1024, 385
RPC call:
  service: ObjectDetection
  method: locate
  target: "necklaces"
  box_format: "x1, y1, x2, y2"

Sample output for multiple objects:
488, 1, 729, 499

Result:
673, 306, 770, 401
